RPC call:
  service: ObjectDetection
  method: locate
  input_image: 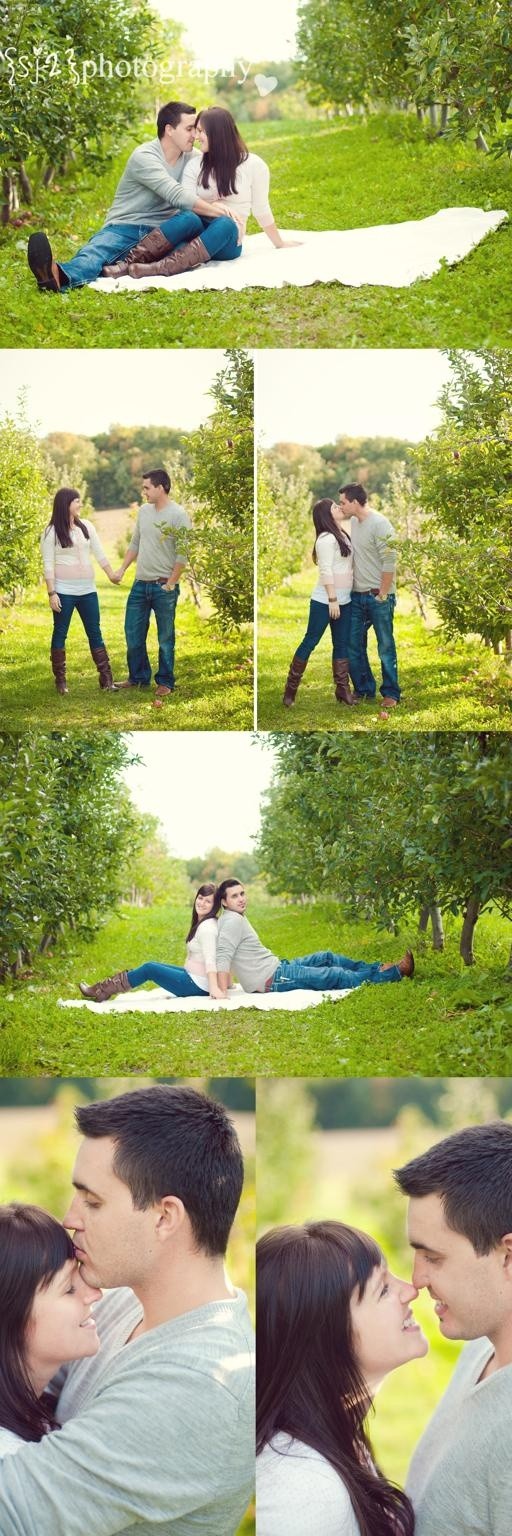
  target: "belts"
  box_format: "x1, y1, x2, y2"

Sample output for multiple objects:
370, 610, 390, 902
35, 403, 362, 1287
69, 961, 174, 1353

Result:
139, 577, 168, 585
361, 587, 380, 595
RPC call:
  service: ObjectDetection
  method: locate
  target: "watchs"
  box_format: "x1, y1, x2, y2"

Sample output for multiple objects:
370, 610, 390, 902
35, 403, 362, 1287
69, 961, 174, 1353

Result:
166, 582, 176, 591
378, 592, 388, 601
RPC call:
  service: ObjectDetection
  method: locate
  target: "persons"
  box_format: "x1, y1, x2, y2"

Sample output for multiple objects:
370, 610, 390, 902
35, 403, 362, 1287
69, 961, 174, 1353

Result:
113, 470, 189, 696
28, 101, 243, 294
78, 882, 229, 1002
337, 483, 401, 707
0, 1088, 512, 1536
102, 106, 303, 279
212, 879, 415, 1000
42, 489, 121, 693
283, 498, 357, 707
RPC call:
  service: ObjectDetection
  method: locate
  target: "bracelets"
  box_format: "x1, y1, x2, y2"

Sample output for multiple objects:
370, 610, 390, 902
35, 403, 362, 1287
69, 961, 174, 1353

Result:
48, 591, 56, 598
328, 597, 337, 602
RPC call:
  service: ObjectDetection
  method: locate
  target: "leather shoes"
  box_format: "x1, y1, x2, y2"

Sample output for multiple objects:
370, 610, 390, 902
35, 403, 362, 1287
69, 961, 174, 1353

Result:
27, 231, 62, 293
154, 685, 172, 698
399, 949, 414, 977
113, 678, 133, 689
381, 697, 397, 709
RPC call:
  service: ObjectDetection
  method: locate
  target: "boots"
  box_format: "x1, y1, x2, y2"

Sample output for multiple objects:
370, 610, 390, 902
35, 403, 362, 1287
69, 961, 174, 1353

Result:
332, 657, 357, 705
79, 969, 131, 1002
100, 228, 172, 278
283, 655, 308, 706
91, 647, 118, 694
128, 235, 211, 279
50, 648, 68, 696
